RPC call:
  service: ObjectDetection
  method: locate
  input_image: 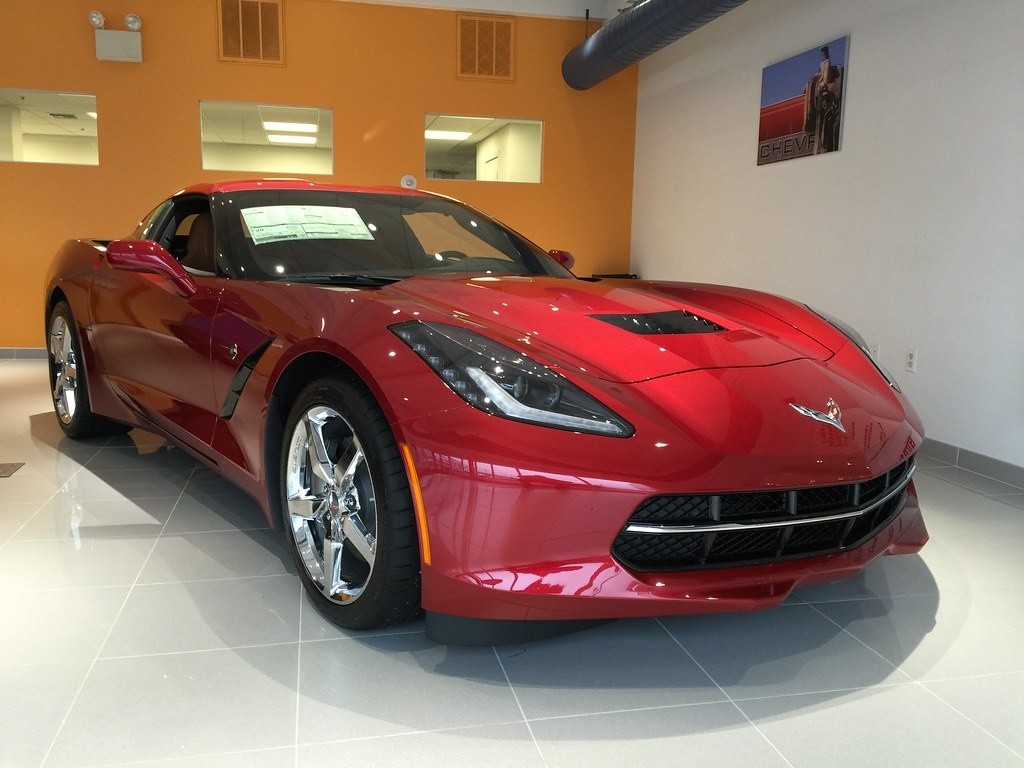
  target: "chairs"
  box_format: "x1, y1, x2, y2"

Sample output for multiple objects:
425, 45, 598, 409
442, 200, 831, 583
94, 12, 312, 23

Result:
178, 214, 216, 275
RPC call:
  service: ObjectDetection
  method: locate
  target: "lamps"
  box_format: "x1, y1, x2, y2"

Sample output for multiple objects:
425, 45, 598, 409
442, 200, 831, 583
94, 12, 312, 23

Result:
89, 11, 143, 63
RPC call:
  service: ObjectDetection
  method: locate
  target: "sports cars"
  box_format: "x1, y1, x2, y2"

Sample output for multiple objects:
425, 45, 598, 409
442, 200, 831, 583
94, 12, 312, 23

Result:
41, 177, 931, 635
